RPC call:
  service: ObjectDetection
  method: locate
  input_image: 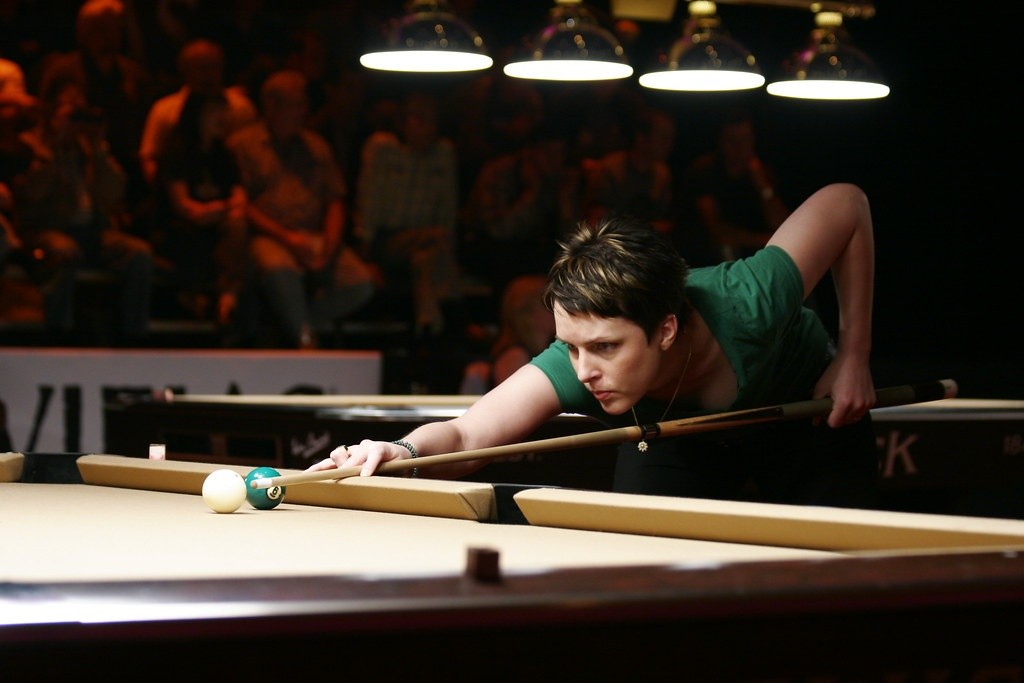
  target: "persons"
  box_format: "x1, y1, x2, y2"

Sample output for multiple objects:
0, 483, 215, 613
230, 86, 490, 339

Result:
310, 183, 880, 510
0, 0, 795, 470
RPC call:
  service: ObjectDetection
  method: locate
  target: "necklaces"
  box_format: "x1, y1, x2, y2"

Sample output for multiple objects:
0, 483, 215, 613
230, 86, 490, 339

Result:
629, 329, 692, 453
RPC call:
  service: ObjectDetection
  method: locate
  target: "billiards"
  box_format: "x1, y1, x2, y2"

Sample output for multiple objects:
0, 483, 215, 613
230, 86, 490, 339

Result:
245, 466, 287, 510
202, 469, 248, 513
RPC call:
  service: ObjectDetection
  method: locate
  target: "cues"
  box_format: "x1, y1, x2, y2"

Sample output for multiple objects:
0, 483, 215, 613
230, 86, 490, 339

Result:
251, 377, 957, 491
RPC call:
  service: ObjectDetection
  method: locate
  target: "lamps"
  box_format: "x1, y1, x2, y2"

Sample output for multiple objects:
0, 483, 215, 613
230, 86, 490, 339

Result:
503, 0, 634, 82
359, 0, 494, 72
636, 0, 765, 92
767, 3, 890, 99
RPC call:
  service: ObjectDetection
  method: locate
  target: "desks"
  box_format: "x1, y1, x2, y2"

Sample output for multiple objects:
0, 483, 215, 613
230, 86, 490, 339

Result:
0, 448, 1024, 683
132, 389, 1023, 521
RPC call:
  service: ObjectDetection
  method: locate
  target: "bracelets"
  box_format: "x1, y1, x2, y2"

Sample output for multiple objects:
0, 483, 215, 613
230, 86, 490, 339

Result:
393, 441, 418, 479
